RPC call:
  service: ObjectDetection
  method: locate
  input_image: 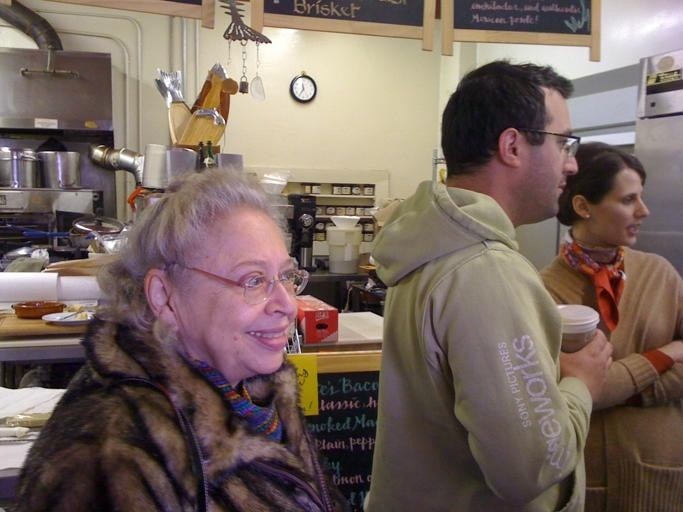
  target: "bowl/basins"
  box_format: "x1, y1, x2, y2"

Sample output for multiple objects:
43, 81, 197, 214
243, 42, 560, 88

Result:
333, 215, 360, 225
14, 300, 65, 317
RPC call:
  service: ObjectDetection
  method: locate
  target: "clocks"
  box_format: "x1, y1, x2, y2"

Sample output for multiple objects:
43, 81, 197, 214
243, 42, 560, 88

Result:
289, 74, 317, 104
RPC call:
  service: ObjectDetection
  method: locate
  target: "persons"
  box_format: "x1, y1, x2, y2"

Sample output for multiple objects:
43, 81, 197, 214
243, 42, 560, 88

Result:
13, 168, 354, 512
367, 60, 616, 511
538, 139, 682, 512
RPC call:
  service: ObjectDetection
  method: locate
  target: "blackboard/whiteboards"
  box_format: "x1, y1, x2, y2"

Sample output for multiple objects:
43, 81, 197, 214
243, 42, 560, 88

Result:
444, 0, 601, 40
285, 353, 383, 510
253, 0, 432, 38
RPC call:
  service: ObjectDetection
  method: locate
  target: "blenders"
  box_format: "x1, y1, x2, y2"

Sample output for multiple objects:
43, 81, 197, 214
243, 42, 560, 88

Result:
291, 194, 318, 273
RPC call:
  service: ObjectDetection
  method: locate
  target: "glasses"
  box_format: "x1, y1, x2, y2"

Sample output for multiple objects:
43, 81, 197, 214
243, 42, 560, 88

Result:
518, 128, 580, 159
165, 262, 310, 305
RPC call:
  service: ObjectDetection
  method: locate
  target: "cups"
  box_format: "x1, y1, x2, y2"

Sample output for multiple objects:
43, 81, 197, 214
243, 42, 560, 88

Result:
555, 303, 599, 349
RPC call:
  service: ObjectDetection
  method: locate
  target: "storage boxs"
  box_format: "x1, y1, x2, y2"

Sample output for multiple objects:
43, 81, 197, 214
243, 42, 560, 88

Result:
296, 294, 338, 346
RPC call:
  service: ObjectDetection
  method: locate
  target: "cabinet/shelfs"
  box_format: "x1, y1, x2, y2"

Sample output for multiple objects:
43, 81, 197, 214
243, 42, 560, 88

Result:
250, 166, 389, 257
